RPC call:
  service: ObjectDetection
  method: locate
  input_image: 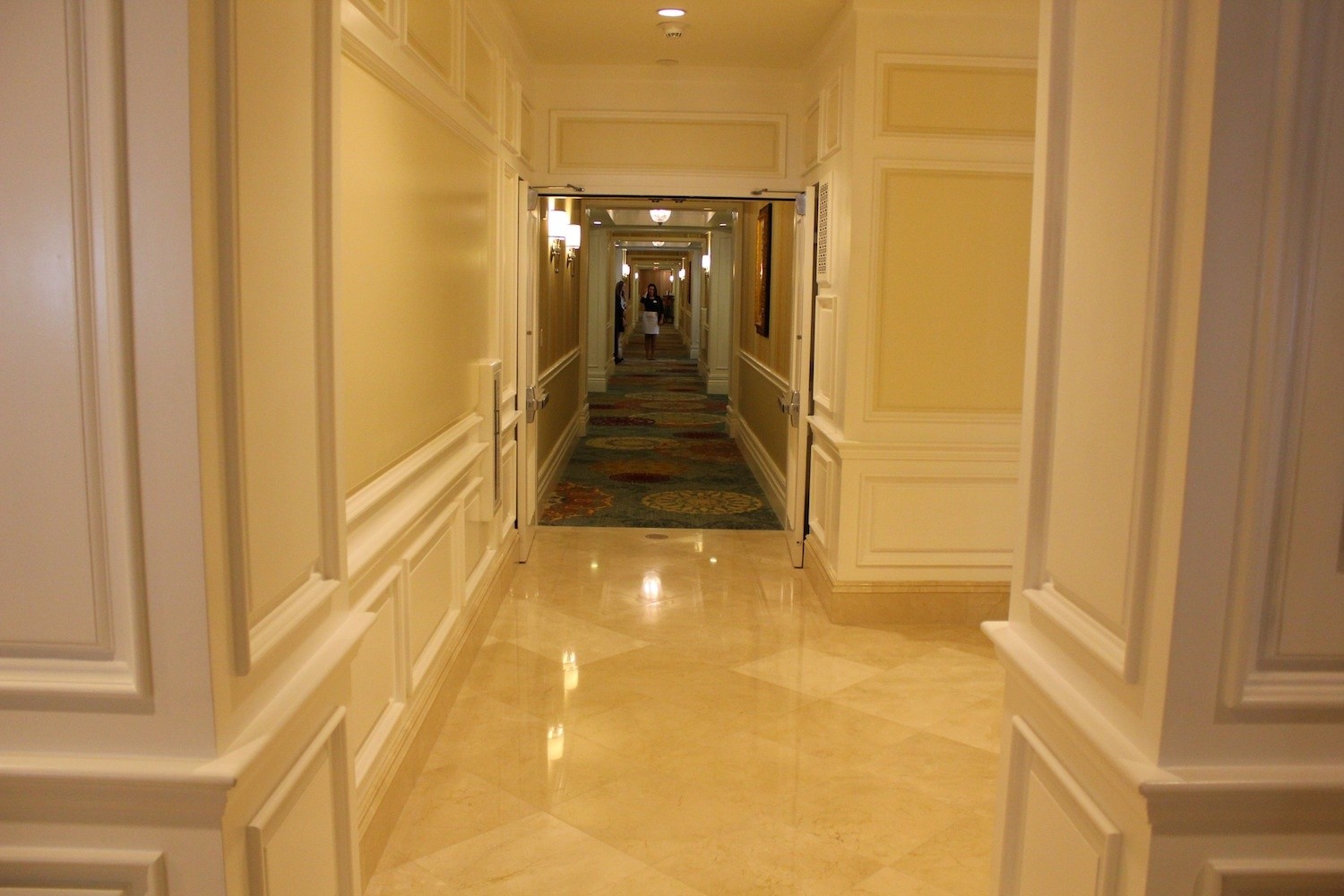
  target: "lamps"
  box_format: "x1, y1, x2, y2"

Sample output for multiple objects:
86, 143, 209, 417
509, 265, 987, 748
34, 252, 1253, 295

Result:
564, 224, 581, 277
649, 209, 671, 223
549, 210, 568, 272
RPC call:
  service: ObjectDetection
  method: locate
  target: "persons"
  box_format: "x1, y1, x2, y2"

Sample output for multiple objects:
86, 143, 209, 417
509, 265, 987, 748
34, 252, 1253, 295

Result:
640, 283, 664, 360
613, 281, 627, 364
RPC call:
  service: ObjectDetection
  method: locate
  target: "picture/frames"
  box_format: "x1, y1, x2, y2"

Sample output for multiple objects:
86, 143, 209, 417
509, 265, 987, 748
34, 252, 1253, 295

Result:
754, 203, 773, 339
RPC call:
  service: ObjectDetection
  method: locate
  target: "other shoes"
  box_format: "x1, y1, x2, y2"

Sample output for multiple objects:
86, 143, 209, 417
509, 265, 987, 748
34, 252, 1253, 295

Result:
614, 357, 623, 364
